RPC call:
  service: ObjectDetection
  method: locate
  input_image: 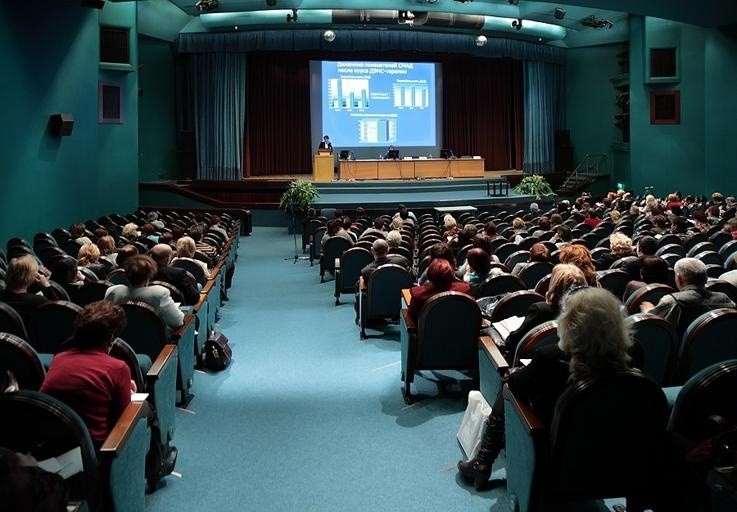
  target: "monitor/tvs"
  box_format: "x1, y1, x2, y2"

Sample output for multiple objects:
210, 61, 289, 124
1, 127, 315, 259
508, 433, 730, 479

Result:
440, 149, 451, 159
388, 149, 399, 160
340, 150, 349, 160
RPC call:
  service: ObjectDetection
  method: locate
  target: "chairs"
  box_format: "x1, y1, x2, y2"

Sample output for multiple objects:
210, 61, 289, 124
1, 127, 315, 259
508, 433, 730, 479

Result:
0, 211, 240, 512
302, 193, 737, 512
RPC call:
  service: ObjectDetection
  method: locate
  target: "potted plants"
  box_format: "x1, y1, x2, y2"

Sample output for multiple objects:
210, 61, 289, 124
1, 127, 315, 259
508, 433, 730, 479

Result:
277, 180, 320, 234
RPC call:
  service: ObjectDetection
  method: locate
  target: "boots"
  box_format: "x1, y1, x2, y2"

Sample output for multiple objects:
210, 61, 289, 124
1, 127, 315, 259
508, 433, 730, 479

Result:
457, 414, 505, 491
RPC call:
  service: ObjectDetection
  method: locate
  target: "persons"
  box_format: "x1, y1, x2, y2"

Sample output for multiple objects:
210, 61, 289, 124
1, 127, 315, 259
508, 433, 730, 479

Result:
109, 245, 138, 272
541, 287, 645, 493
146, 244, 199, 306
317, 135, 332, 149
92, 228, 108, 242
95, 235, 116, 264
49, 257, 92, 292
171, 236, 211, 279
303, 191, 733, 345
105, 255, 185, 337
622, 256, 670, 304
118, 211, 234, 300
75, 243, 100, 269
1, 254, 59, 323
38, 299, 178, 487
67, 223, 92, 245
641, 256, 734, 335
381, 146, 395, 159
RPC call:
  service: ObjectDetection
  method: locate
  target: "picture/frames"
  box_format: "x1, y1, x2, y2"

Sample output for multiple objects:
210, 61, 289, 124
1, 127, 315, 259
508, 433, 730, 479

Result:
649, 89, 681, 125
644, 43, 681, 84
97, 79, 124, 124
96, 20, 134, 72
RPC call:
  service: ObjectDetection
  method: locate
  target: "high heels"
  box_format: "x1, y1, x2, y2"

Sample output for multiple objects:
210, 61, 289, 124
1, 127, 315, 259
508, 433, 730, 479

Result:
147, 447, 178, 491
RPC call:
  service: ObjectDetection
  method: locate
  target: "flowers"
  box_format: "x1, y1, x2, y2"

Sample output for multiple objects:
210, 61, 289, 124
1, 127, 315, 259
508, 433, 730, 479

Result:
512, 174, 553, 195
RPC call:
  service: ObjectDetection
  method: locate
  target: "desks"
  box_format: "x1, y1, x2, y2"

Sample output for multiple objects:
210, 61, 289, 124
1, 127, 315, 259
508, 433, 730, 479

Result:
338, 158, 484, 181
485, 178, 510, 197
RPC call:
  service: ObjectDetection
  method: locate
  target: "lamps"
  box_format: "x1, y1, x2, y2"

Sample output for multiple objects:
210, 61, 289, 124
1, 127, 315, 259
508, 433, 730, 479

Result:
285, 9, 301, 20
324, 25, 335, 42
513, 18, 525, 33
554, 6, 566, 19
475, 27, 488, 49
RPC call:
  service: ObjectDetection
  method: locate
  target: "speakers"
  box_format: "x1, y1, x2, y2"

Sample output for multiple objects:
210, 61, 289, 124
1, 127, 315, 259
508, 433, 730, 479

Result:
52, 112, 75, 136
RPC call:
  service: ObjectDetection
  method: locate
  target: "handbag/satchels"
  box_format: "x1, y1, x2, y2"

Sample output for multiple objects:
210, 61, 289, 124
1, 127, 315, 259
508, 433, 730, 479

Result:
205, 330, 232, 371
